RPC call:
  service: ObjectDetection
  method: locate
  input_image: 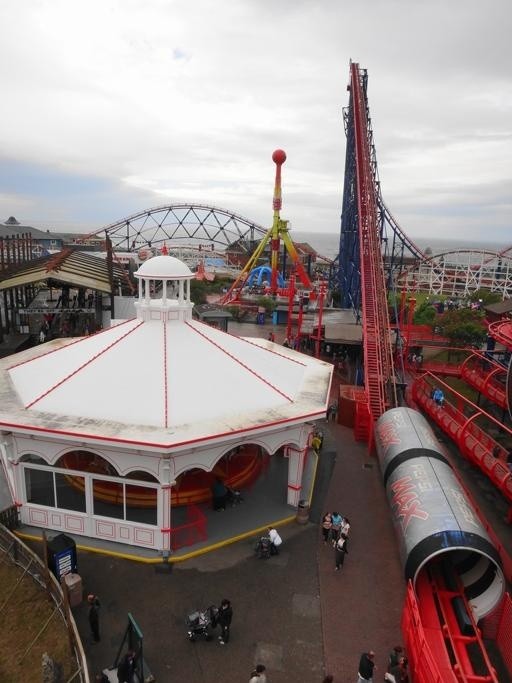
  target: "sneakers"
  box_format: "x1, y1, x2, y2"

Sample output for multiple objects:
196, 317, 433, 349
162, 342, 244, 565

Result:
218, 636, 224, 645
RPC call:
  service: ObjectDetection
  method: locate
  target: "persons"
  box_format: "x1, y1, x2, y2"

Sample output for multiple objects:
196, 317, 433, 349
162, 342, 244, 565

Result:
331, 403, 337, 422
268, 526, 282, 555
407, 351, 425, 369
383, 655, 408, 683
430, 385, 436, 399
249, 664, 266, 683
321, 510, 350, 570
357, 649, 377, 683
38, 292, 94, 344
387, 646, 403, 668
433, 387, 444, 404
117, 649, 137, 682
87, 602, 101, 644
269, 331, 349, 372
326, 404, 331, 422
211, 599, 233, 644
210, 476, 227, 512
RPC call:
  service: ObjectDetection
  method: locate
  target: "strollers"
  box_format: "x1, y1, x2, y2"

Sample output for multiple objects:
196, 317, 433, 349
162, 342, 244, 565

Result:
184, 604, 220, 643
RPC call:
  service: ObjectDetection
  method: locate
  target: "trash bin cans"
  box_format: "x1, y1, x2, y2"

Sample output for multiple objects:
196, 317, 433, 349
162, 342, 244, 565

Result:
296, 499, 310, 525
61, 573, 83, 609
